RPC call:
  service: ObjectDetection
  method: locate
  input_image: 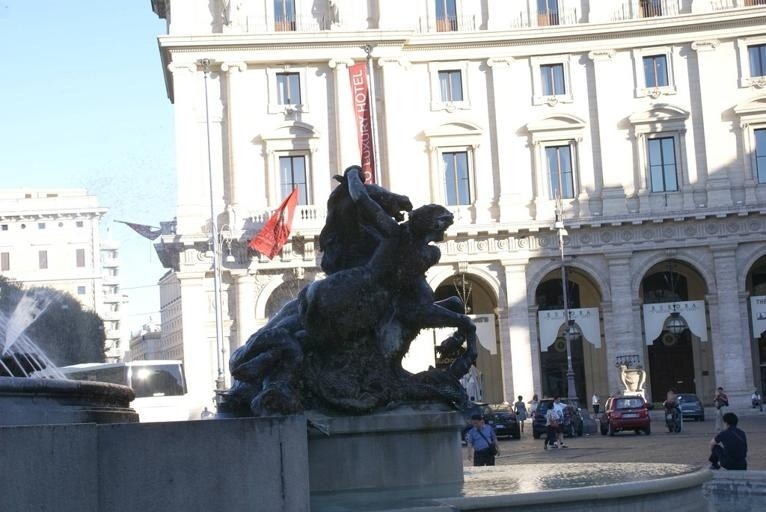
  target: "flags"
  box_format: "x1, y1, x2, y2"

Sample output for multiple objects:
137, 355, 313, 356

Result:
246, 189, 299, 260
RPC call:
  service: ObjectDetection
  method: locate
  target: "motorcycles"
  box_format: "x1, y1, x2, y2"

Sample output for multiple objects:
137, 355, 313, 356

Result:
664, 395, 684, 432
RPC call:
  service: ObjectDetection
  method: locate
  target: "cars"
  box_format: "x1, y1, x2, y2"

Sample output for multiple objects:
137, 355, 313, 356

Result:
489, 404, 521, 439
680, 393, 704, 422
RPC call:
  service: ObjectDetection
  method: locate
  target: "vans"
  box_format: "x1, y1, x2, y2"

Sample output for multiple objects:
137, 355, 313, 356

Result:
28, 357, 192, 424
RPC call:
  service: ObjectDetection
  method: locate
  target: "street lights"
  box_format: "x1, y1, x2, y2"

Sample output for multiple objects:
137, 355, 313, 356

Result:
554, 206, 582, 411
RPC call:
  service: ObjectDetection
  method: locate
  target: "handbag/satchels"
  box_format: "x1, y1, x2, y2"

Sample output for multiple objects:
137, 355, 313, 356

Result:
550, 420, 559, 428
489, 443, 497, 456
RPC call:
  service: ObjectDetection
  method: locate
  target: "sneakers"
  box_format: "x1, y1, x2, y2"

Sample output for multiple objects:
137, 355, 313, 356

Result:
710, 464, 721, 470
544, 442, 568, 449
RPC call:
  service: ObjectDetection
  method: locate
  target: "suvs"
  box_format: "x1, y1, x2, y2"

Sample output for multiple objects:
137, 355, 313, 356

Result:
533, 397, 583, 439
461, 401, 496, 444
599, 394, 652, 436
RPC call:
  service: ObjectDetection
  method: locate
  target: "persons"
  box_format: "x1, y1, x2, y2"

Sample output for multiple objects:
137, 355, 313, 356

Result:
543, 402, 560, 449
528, 394, 539, 422
553, 395, 572, 448
240, 167, 471, 397
467, 413, 501, 466
592, 392, 601, 413
662, 388, 680, 406
514, 395, 528, 432
752, 391, 764, 411
707, 412, 748, 471
714, 387, 729, 430
